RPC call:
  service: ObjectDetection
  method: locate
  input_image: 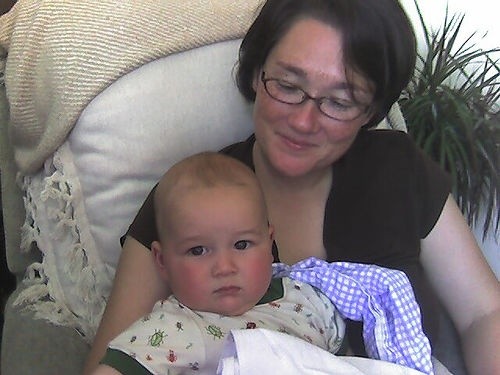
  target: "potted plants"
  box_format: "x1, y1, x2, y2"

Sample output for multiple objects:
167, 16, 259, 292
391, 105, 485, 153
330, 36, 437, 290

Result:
346, 0, 500, 359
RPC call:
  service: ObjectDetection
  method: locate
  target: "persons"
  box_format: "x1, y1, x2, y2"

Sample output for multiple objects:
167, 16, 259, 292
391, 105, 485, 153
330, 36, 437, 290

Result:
81, 0, 500, 375
90, 152, 449, 375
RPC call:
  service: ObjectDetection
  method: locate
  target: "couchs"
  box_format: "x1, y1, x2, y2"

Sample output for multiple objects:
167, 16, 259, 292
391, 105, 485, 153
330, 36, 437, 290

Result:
0, 0, 469, 374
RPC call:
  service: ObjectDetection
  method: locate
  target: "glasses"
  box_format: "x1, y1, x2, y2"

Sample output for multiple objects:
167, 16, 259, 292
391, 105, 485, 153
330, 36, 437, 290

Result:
261, 71, 376, 122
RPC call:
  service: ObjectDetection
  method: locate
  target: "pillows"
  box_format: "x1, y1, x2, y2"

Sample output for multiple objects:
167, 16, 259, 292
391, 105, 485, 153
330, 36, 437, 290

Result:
0, 0, 390, 345
